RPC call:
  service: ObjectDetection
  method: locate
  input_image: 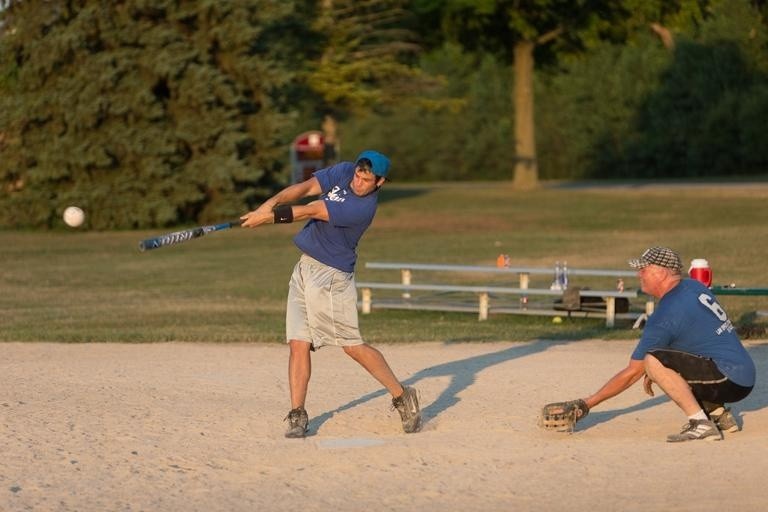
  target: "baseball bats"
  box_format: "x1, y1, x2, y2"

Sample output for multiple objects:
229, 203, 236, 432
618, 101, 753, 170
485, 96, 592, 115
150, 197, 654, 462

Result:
139, 219, 248, 252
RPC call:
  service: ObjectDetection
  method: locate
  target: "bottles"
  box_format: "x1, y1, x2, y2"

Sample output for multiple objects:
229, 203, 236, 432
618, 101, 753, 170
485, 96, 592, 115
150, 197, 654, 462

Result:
562, 260, 569, 286
617, 279, 624, 294
553, 261, 562, 286
498, 255, 510, 269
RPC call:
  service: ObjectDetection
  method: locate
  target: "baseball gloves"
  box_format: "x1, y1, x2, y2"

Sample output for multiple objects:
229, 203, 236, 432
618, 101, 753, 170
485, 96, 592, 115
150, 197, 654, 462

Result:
539, 399, 588, 434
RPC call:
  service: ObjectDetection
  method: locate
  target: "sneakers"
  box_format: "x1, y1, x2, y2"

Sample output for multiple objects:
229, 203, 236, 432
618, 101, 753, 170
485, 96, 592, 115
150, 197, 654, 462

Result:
708, 406, 739, 434
666, 416, 723, 443
283, 406, 311, 439
390, 384, 422, 433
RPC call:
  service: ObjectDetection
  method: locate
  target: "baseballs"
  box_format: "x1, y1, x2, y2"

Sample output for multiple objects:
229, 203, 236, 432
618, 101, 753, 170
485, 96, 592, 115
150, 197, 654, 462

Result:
63, 205, 84, 226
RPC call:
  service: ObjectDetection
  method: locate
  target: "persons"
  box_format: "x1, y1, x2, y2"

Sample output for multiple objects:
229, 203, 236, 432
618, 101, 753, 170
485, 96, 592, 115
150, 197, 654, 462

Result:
239, 148, 421, 443
542, 248, 756, 443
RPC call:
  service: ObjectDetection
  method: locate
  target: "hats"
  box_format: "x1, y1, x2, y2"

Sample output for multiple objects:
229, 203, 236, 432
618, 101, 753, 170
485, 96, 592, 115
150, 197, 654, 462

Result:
627, 246, 683, 271
352, 150, 391, 181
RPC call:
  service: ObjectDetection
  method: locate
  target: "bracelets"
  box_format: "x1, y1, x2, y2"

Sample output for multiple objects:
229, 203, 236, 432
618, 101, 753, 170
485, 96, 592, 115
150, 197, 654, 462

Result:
273, 204, 292, 224
